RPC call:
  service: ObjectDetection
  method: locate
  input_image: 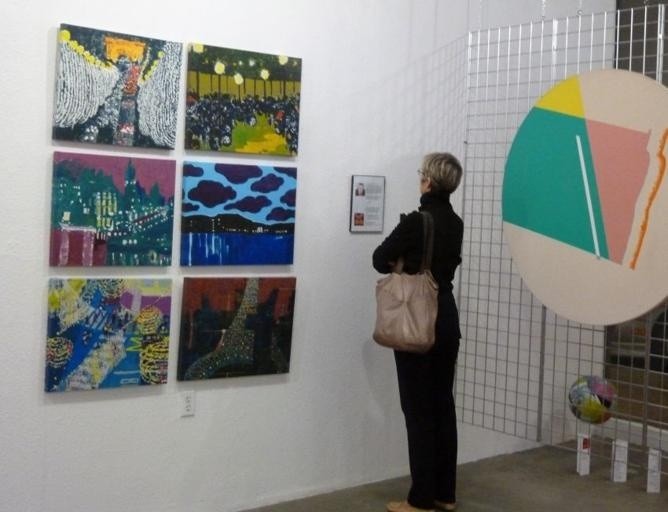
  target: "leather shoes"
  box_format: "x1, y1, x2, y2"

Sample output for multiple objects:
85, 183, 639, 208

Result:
386, 499, 435, 512
436, 501, 457, 510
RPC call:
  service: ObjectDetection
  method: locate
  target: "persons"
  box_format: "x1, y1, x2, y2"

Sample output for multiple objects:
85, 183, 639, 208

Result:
372, 153, 464, 512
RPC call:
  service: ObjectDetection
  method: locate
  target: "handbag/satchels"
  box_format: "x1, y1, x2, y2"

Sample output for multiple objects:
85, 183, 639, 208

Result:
371, 272, 440, 352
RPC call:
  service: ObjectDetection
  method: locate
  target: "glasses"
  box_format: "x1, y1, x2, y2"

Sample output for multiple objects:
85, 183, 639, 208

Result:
417, 168, 429, 179
